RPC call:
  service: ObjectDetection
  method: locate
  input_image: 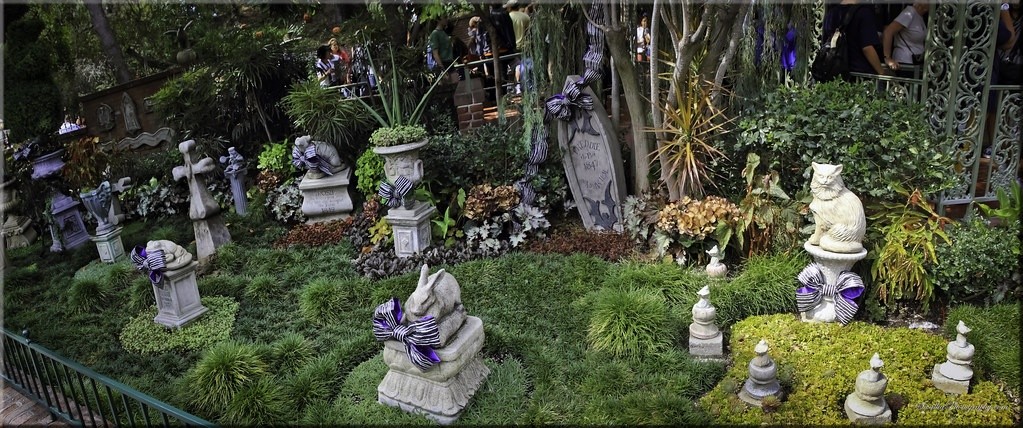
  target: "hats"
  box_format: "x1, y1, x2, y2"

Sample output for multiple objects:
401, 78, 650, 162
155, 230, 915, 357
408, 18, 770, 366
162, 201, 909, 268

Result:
469, 16, 481, 27
279, 32, 302, 46
503, 0, 519, 8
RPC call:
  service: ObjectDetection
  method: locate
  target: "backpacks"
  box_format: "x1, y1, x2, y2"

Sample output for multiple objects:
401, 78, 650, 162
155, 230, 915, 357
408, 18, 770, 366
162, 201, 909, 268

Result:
811, 43, 847, 81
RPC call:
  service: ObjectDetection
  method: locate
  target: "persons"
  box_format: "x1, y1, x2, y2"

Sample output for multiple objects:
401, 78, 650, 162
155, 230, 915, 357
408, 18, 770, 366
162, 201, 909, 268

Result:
316, 37, 350, 88
636, 14, 651, 61
427, 0, 536, 80
59, 114, 85, 134
848, 0, 1023, 158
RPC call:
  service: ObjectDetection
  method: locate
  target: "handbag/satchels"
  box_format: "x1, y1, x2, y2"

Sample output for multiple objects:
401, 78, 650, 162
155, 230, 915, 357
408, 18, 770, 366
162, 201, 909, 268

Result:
912, 54, 925, 65
427, 30, 438, 71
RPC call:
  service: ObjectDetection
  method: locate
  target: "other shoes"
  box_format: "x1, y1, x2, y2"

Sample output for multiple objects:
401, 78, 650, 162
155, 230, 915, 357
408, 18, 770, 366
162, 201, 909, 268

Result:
983, 146, 991, 158
